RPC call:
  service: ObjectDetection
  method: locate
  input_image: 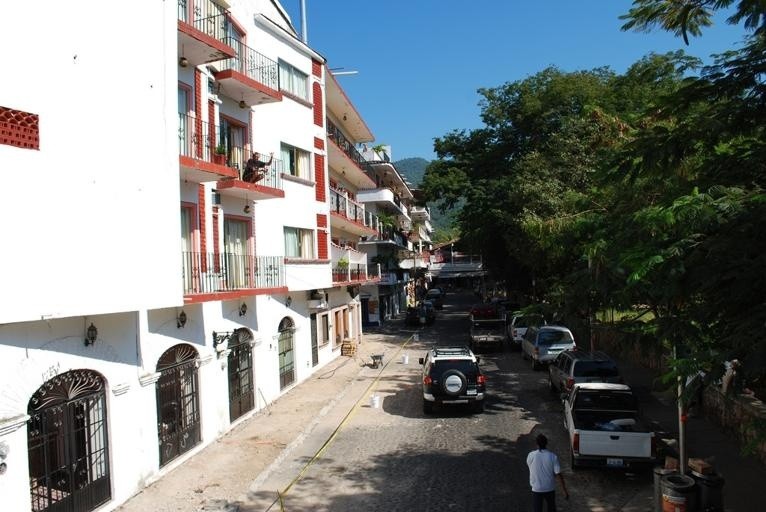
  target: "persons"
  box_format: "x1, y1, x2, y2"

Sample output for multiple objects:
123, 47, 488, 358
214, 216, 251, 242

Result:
526, 433, 568, 512
242, 152, 273, 183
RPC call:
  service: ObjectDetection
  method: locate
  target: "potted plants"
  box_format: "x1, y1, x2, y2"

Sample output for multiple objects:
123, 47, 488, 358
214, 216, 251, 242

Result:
337, 257, 349, 281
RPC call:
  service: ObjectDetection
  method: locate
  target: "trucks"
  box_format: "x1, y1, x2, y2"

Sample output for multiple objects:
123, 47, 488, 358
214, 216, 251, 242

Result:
467, 301, 510, 352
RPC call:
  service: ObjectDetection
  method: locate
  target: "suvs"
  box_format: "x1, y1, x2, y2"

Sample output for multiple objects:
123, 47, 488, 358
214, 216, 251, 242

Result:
417, 347, 487, 414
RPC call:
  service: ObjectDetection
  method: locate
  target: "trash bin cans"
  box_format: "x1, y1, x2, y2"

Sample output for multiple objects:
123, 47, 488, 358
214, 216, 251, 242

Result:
654, 464, 724, 512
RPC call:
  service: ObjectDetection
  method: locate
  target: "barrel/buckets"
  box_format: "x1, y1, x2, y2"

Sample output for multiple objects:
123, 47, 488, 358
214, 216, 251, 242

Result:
661, 474, 694, 512
403, 355, 408, 364
370, 395, 379, 408
654, 467, 677, 511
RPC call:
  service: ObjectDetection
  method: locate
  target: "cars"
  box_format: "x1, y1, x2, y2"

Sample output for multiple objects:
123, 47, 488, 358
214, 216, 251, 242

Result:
511, 308, 627, 394
404, 283, 446, 327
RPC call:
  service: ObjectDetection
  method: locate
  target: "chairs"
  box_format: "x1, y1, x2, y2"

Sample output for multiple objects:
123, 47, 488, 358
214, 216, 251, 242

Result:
342, 339, 356, 357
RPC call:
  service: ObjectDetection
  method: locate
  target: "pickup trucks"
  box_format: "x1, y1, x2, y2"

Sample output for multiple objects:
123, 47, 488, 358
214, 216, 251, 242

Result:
562, 382, 658, 473
